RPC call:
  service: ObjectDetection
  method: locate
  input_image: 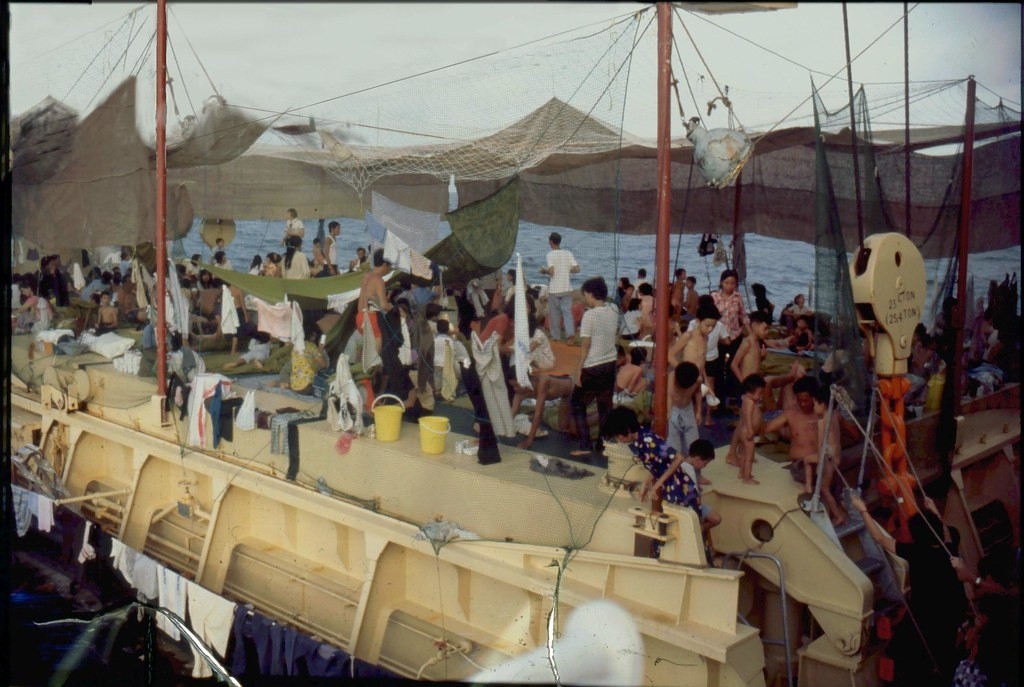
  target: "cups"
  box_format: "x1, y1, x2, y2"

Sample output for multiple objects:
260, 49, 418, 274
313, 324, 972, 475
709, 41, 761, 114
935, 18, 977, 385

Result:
701, 384, 708, 397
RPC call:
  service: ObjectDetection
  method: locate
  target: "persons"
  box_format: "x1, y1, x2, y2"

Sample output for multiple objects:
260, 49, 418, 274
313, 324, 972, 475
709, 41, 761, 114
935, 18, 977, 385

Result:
12, 209, 1024, 687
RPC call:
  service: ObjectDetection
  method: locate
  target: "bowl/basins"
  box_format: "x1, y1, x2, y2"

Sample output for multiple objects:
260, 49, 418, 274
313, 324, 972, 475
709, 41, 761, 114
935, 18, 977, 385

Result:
706, 395, 720, 406
60, 343, 87, 356
463, 447, 479, 456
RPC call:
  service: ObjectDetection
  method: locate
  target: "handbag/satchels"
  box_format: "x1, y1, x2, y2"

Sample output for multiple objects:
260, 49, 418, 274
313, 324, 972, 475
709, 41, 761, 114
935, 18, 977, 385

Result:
925, 366, 945, 409
716, 239, 726, 267
698, 233, 717, 256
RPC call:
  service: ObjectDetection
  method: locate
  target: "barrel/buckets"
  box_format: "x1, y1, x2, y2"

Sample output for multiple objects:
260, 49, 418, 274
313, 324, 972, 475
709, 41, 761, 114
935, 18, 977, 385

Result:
418, 416, 451, 454
371, 394, 406, 441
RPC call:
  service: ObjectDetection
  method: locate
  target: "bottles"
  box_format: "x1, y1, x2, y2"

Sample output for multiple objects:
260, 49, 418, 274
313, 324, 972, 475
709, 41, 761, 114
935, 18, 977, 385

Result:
371, 424, 376, 439
